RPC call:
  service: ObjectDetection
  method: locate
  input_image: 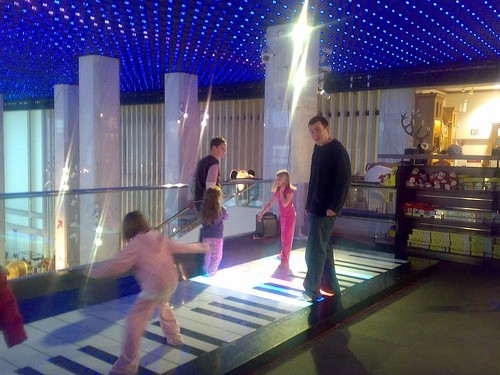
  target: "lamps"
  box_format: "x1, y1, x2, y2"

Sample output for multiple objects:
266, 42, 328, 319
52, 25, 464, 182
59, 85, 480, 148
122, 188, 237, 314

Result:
319, 47, 333, 100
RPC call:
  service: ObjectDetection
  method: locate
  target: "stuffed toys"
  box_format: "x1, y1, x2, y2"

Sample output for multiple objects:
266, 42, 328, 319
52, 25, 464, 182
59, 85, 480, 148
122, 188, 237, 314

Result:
405, 166, 458, 190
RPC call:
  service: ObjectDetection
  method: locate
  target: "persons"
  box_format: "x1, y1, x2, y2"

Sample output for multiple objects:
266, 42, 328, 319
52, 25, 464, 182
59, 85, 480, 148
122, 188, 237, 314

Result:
189, 136, 227, 275
433, 149, 451, 166
83, 210, 210, 375
257, 169, 297, 271
297, 115, 352, 302
198, 187, 230, 277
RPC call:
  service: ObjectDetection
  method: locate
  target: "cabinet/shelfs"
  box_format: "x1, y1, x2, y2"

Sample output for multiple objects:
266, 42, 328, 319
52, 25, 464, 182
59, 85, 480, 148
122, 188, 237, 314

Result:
394, 166, 500, 267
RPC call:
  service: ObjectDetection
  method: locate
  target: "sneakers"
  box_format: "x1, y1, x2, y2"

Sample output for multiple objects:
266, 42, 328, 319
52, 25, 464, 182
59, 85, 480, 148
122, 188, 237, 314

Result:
298, 294, 312, 301
167, 338, 183, 345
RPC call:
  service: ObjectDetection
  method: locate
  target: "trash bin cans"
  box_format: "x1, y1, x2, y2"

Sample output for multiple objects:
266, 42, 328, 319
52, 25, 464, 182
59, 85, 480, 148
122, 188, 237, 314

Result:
256, 213, 278, 238
